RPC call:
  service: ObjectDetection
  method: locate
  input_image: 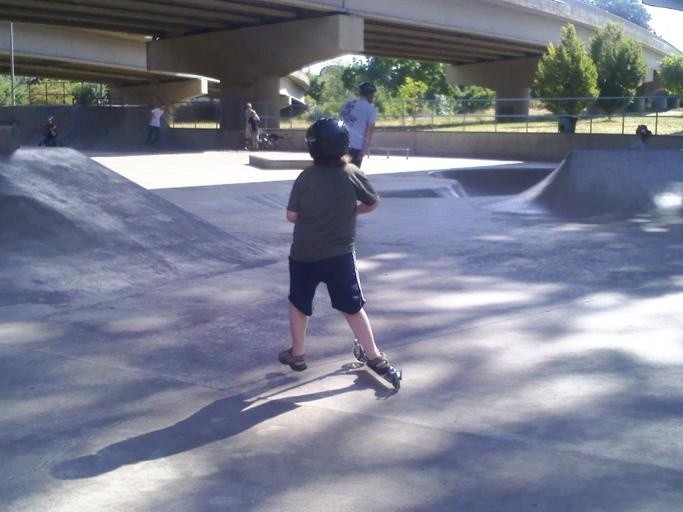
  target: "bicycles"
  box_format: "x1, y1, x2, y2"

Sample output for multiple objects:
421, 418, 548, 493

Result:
243, 130, 283, 151
37, 130, 65, 147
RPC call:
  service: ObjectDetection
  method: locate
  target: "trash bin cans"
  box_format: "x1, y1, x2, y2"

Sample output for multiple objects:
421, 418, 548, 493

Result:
557, 115, 577, 133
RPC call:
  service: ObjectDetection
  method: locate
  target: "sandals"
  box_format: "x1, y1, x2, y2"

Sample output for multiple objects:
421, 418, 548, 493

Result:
366, 352, 389, 373
279, 347, 307, 371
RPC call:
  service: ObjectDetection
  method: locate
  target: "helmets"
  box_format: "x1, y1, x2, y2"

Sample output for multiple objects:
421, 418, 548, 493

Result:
306, 118, 350, 159
360, 82, 375, 93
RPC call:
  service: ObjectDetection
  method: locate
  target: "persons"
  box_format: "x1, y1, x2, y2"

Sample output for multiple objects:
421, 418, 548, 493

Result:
243, 103, 260, 153
338, 81, 378, 169
144, 103, 166, 148
276, 115, 401, 384
39, 116, 57, 147
